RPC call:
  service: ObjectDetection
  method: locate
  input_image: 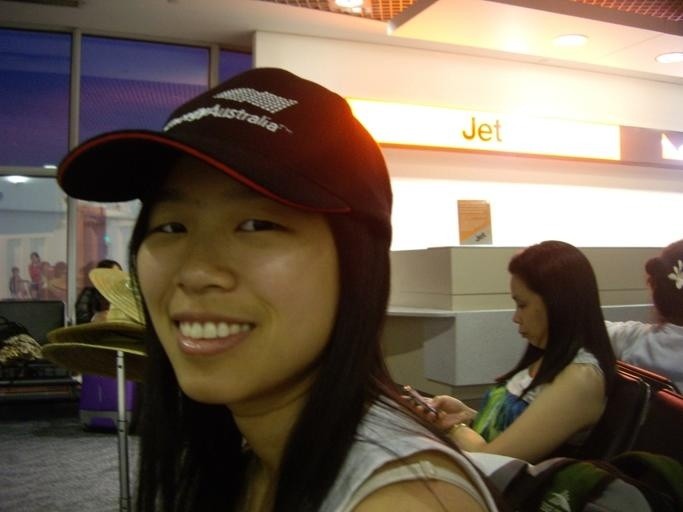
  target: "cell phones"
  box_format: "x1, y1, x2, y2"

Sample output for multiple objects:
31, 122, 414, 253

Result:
403, 383, 437, 414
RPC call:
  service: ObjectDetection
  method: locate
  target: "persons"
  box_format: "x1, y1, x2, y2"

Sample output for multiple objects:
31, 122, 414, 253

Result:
54, 66, 501, 512
402, 240, 622, 512
9, 252, 67, 299
603, 238, 683, 394
73, 259, 122, 324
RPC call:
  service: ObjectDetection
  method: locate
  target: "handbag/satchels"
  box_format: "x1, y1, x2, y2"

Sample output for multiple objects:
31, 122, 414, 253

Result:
1, 316, 47, 380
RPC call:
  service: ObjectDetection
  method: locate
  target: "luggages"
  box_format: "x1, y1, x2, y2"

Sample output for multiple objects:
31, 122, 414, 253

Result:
79, 370, 135, 433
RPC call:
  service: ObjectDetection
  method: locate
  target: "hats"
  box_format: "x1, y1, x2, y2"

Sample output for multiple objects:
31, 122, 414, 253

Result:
55, 67, 393, 220
41, 267, 148, 378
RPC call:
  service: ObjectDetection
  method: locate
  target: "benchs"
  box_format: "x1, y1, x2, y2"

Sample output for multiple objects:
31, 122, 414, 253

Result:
0, 300, 81, 406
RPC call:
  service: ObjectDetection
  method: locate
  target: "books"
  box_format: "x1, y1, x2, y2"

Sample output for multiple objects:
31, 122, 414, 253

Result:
457, 199, 493, 245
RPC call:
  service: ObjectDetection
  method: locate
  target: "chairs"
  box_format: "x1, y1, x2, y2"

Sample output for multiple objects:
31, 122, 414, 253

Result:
581, 359, 683, 512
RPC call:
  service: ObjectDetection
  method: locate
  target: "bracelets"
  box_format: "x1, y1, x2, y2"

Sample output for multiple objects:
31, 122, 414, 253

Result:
445, 422, 467, 438
